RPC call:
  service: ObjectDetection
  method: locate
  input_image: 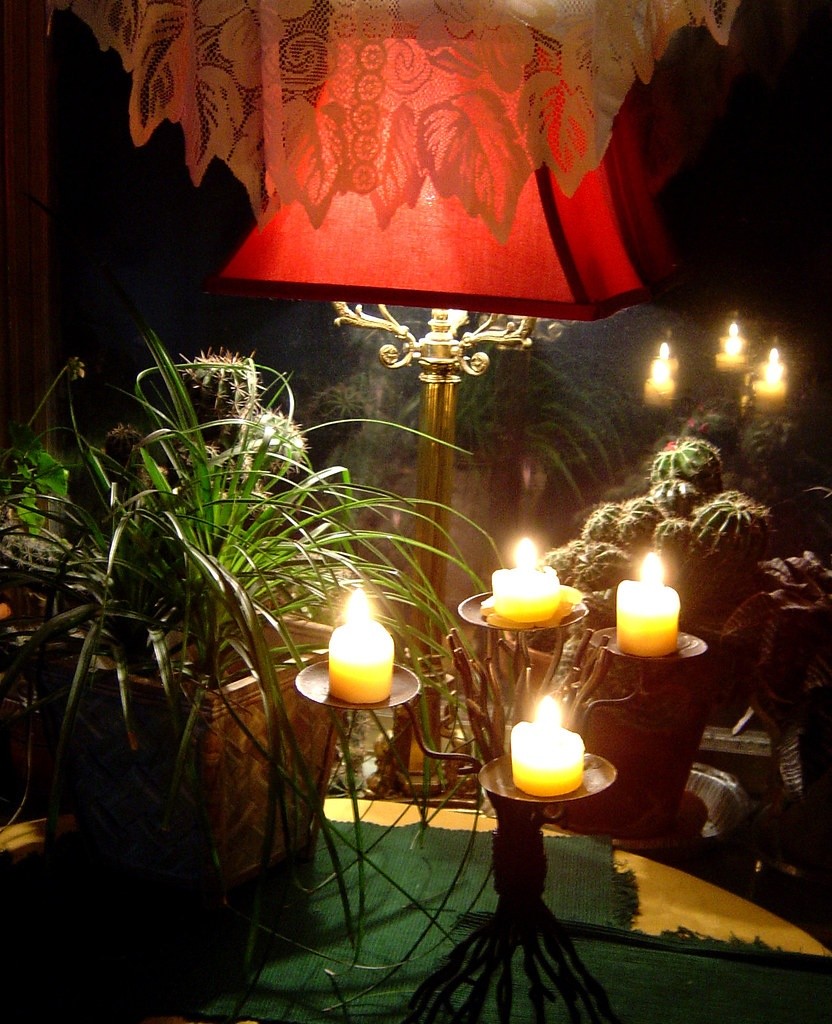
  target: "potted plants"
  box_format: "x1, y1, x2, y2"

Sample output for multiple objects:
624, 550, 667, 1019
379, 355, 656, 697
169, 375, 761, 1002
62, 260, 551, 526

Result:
325, 325, 642, 593
1, 327, 340, 911
504, 395, 803, 835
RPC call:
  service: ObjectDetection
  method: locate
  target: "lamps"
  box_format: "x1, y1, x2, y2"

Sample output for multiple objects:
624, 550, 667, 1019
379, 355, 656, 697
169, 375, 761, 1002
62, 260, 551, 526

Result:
200, 0, 691, 810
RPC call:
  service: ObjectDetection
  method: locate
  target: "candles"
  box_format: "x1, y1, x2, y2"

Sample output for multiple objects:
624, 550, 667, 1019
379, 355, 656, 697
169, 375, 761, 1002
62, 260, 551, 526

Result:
753, 347, 788, 413
480, 530, 571, 628
327, 578, 396, 704
510, 682, 585, 797
716, 321, 746, 372
643, 343, 679, 405
617, 544, 681, 656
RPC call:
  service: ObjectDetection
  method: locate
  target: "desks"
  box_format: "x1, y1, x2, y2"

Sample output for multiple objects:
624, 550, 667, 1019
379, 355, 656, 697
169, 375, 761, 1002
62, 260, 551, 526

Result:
0, 798, 832, 1024
